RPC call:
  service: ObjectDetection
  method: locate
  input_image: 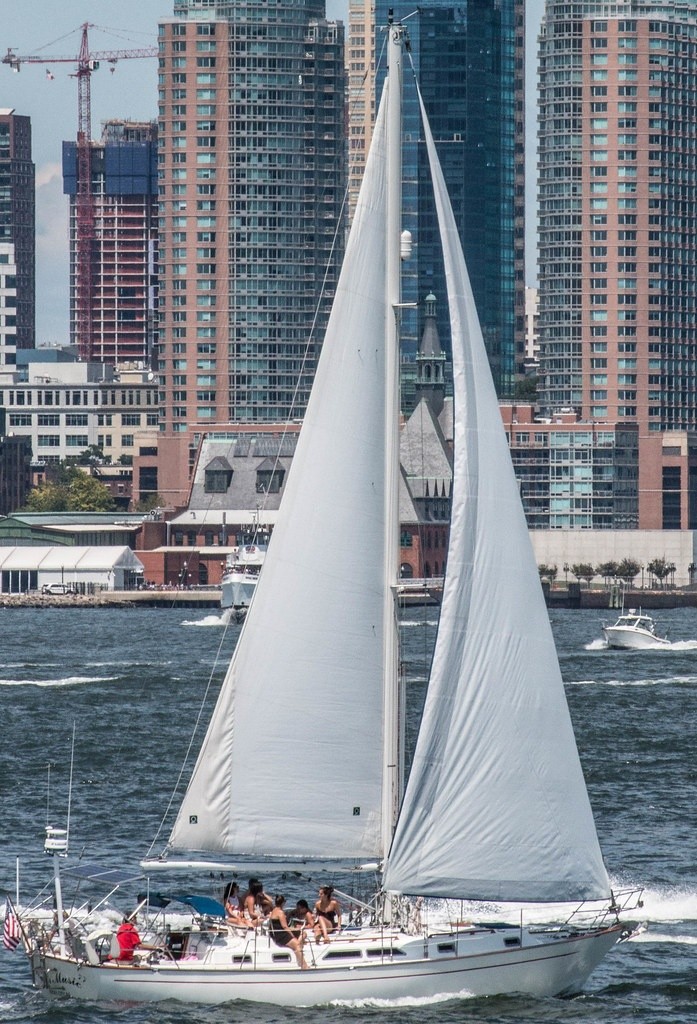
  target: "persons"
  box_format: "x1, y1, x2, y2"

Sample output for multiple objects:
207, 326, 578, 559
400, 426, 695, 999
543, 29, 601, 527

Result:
104, 910, 163, 964
221, 875, 343, 968
45, 911, 75, 952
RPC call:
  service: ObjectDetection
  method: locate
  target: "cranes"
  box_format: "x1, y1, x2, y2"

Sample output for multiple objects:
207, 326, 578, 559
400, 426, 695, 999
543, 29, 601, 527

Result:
2, 21, 160, 364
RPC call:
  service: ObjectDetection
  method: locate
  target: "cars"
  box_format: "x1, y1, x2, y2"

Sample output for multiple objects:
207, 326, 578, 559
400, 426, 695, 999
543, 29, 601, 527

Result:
44, 582, 75, 595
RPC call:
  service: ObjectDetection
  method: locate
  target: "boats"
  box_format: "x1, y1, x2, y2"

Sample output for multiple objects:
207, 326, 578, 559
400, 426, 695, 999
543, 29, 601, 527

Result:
220, 504, 269, 622
599, 605, 672, 650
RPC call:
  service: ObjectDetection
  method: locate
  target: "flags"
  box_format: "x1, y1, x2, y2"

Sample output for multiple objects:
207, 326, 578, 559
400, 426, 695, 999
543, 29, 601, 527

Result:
2, 897, 24, 951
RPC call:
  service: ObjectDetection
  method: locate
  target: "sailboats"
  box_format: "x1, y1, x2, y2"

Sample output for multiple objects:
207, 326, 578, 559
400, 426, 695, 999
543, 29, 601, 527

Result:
0, 6, 645, 1010
